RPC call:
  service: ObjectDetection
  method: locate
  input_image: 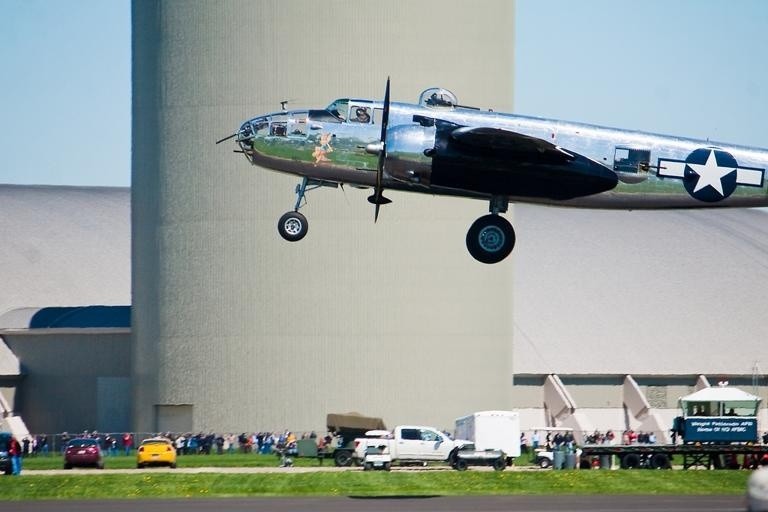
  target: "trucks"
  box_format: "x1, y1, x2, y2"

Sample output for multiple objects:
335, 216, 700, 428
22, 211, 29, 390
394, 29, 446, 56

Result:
449, 409, 523, 470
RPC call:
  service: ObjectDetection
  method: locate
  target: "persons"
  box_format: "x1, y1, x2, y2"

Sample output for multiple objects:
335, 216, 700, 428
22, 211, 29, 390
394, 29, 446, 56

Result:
58, 431, 71, 456
519, 429, 656, 452
726, 408, 738, 416
121, 432, 133, 456
152, 432, 215, 455
216, 432, 235, 455
694, 406, 704, 416
23, 434, 47, 458
238, 431, 296, 467
76, 431, 120, 456
350, 107, 369, 123
9, 436, 21, 475
301, 430, 322, 451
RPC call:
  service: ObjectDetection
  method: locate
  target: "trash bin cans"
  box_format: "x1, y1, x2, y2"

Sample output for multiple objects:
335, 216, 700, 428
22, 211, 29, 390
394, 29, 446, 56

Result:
553, 451, 577, 470
599, 455, 610, 470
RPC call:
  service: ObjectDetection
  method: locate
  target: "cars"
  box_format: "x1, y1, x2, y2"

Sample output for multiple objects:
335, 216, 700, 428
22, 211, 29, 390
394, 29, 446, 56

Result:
0, 432, 24, 479
134, 438, 180, 469
64, 438, 104, 470
537, 445, 608, 469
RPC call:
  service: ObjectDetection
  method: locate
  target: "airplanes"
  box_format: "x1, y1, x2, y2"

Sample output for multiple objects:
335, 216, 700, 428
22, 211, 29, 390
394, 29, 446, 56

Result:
210, 77, 767, 266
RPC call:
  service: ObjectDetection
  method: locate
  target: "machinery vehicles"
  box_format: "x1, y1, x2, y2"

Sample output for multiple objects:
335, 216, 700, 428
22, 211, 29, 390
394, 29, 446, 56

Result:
318, 412, 397, 469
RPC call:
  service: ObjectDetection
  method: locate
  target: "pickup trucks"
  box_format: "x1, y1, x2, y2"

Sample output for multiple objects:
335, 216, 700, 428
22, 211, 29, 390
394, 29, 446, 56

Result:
348, 425, 474, 469
